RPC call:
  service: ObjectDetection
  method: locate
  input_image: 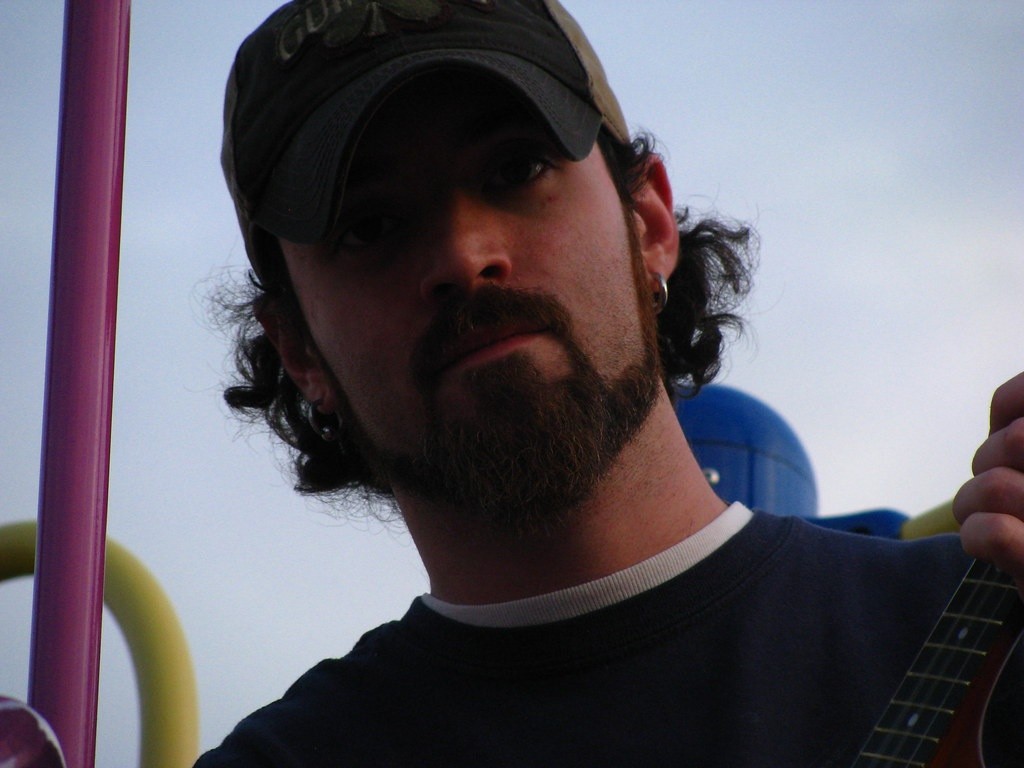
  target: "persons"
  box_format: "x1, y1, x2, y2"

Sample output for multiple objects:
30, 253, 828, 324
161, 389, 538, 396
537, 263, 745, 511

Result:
194, 0, 1024, 768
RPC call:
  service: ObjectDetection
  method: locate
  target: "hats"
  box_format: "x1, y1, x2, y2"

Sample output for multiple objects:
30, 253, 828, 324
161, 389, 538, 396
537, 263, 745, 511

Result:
220, 0, 633, 295
